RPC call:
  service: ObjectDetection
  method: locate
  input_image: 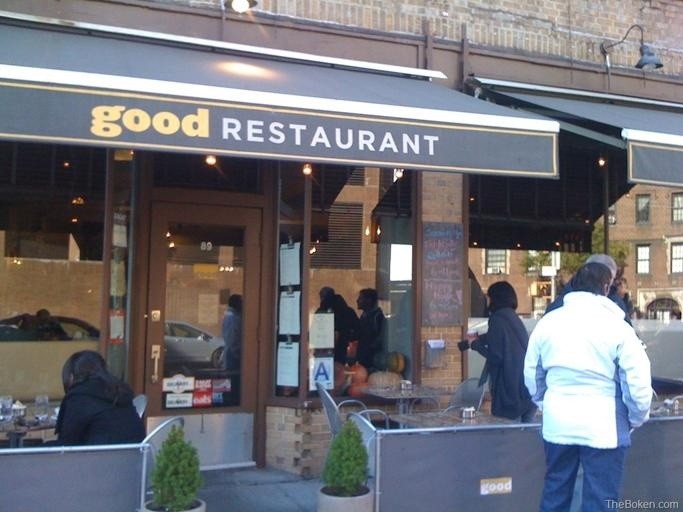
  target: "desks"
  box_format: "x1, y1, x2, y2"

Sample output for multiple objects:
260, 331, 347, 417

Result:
359, 384, 455, 428
388, 410, 518, 428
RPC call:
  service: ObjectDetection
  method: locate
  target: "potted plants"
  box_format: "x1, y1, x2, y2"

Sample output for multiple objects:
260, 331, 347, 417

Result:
317, 417, 375, 512
142, 422, 206, 512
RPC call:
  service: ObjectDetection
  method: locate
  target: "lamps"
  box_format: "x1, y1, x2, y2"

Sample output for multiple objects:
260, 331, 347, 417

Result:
302, 164, 312, 175
601, 25, 663, 71
227, 0, 257, 13
206, 155, 218, 165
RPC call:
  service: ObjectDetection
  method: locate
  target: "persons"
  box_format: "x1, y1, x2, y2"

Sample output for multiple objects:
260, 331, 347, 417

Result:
463, 281, 537, 424
39, 350, 145, 445
220, 293, 241, 405
35, 308, 74, 340
545, 253, 634, 328
617, 277, 637, 320
311, 285, 359, 365
524, 262, 652, 511
346, 286, 388, 378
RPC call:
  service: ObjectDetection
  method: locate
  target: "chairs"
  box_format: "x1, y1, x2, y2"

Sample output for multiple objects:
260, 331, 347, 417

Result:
345, 413, 377, 488
651, 390, 658, 401
134, 395, 147, 417
140, 415, 185, 505
672, 395, 683, 404
442, 378, 485, 414
520, 407, 539, 431
314, 377, 389, 437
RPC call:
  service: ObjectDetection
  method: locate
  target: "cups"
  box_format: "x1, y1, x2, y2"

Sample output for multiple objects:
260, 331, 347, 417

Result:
33, 394, 50, 424
0, 394, 14, 423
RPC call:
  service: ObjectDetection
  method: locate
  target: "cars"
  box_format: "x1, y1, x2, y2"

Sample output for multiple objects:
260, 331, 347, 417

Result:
0, 313, 225, 402
